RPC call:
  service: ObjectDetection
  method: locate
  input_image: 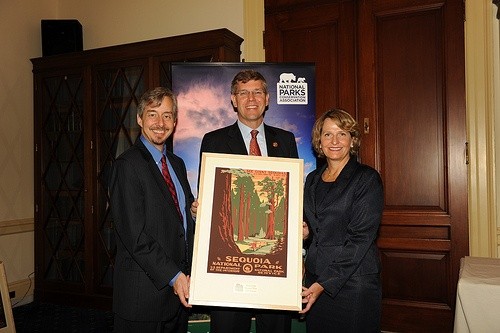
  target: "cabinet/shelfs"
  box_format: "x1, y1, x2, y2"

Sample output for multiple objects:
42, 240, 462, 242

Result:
263, 0, 469, 333
30, 28, 245, 333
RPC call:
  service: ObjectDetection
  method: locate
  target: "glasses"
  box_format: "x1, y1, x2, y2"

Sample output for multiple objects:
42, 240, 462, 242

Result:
235, 89, 266, 97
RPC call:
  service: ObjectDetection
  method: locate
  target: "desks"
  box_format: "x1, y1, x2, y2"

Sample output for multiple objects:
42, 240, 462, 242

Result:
453, 257, 500, 333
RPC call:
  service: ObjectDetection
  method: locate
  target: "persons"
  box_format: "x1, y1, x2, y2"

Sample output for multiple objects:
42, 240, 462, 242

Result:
299, 107, 384, 333
107, 86, 199, 333
197, 68, 300, 333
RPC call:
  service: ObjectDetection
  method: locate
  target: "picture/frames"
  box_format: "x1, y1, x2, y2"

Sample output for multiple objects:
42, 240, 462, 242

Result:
187, 152, 304, 311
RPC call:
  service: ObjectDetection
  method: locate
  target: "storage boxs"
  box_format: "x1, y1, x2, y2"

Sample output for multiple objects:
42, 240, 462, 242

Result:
40, 18, 83, 57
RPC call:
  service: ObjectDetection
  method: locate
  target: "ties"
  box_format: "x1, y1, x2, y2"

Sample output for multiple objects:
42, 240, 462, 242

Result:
161, 155, 184, 224
249, 130, 261, 156
